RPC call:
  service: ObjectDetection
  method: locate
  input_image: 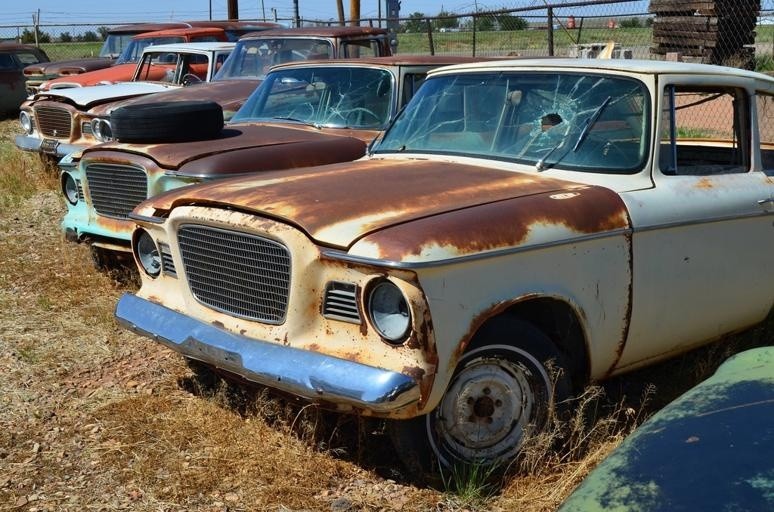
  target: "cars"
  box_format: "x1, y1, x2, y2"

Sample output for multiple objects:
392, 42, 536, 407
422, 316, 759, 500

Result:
108, 56, 774, 482
